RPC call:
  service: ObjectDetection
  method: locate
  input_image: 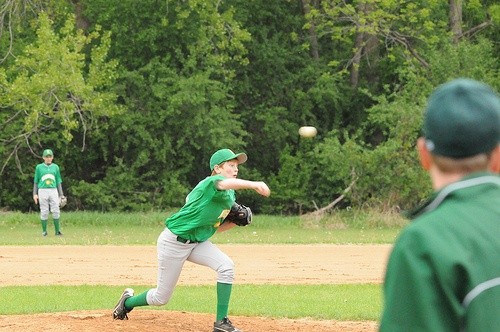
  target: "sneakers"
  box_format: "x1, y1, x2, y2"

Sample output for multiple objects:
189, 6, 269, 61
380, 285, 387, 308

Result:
112, 288, 134, 320
214, 318, 240, 332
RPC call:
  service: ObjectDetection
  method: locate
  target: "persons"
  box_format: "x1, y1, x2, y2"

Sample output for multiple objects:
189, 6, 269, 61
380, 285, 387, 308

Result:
375, 77, 500, 332
32, 149, 64, 237
112, 148, 271, 332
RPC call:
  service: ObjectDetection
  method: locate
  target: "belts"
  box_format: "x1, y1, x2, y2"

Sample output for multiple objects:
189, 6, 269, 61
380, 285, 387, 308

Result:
177, 237, 194, 244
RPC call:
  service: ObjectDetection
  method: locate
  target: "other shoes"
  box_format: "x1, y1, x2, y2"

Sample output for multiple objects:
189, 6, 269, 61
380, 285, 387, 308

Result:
56, 232, 62, 236
43, 231, 48, 236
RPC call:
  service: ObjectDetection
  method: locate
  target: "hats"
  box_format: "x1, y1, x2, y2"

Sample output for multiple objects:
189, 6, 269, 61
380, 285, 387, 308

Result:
422, 77, 500, 157
43, 149, 53, 158
209, 149, 247, 170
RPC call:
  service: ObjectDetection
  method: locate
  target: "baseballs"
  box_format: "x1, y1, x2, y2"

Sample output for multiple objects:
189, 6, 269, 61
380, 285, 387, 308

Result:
298, 127, 318, 138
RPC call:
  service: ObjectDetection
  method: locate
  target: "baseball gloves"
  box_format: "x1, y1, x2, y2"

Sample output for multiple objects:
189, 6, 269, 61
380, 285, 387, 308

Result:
59, 196, 67, 208
228, 203, 251, 227
